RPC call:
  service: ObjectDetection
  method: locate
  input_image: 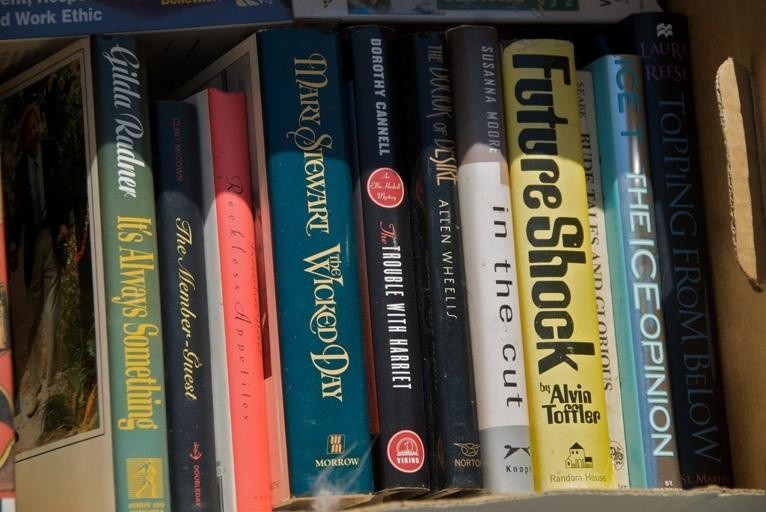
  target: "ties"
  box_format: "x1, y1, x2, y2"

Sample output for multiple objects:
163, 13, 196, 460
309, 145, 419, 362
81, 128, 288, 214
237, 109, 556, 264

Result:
33, 156, 42, 225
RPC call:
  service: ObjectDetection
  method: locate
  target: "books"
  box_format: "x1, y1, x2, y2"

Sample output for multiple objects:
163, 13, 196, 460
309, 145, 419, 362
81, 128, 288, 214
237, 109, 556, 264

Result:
13, 37, 172, 512
193, 89, 275, 512
344, 21, 432, 498
587, 53, 682, 493
446, 24, 534, 498
578, 68, 630, 492
190, 24, 372, 502
406, 28, 484, 495
500, 40, 615, 493
150, 96, 221, 512
626, 9, 734, 495
